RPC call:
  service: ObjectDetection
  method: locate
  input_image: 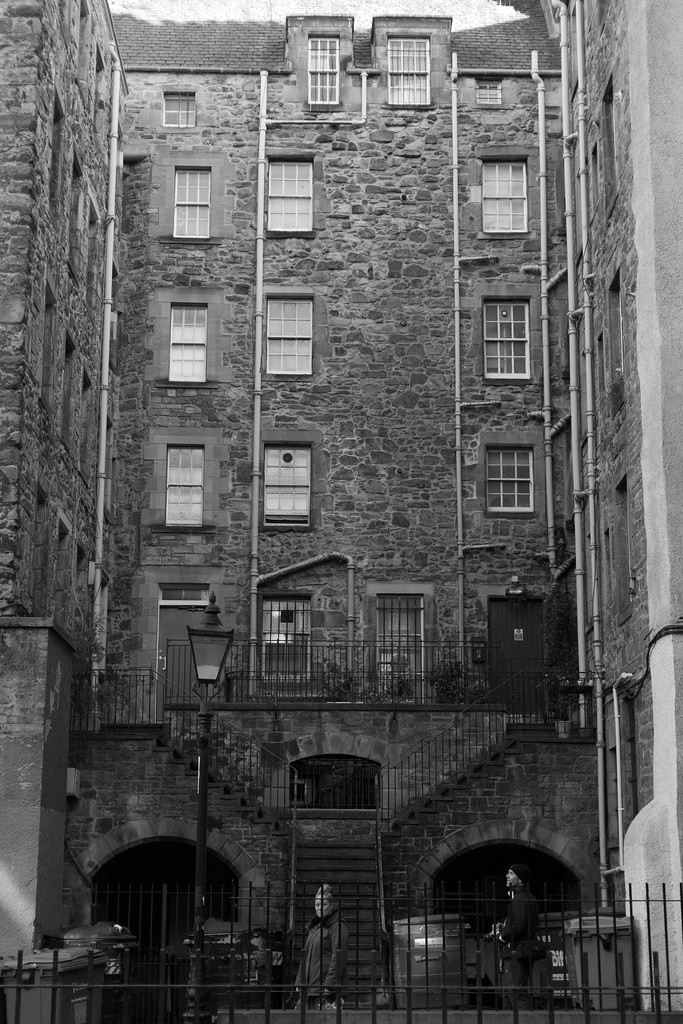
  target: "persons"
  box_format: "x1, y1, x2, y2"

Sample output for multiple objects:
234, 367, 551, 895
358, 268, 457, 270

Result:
297, 883, 349, 1009
500, 864, 541, 1012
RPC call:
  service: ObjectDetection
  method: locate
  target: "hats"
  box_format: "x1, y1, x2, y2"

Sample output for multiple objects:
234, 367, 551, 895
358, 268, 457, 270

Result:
510, 864, 531, 884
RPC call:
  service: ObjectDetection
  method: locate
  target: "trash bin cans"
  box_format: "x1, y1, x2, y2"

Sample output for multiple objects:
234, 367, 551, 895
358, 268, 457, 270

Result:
562, 915, 636, 1012
3, 946, 111, 1024
43, 921, 141, 1024
527, 910, 579, 999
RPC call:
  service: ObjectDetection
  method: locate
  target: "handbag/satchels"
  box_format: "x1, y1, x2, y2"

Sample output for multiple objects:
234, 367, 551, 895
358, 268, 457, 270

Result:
519, 939, 547, 959
316, 993, 345, 1010
